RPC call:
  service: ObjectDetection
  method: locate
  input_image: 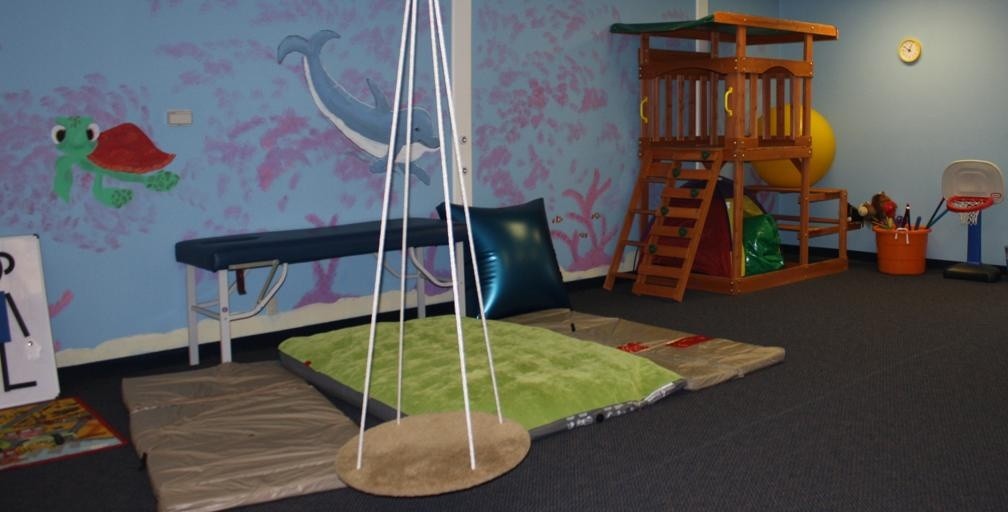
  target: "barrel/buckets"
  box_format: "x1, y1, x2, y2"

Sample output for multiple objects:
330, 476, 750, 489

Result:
871, 222, 933, 276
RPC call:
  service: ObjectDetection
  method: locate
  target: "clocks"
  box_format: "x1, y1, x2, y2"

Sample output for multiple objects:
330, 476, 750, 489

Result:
895, 37, 924, 67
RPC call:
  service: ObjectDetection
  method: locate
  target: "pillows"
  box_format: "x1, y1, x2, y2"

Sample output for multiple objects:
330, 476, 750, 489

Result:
433, 197, 574, 323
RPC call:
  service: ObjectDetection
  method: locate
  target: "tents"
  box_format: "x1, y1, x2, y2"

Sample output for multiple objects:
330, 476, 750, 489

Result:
632, 175, 785, 278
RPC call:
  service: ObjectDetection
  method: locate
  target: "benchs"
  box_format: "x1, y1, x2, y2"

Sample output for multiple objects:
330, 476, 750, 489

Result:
175, 216, 470, 366
